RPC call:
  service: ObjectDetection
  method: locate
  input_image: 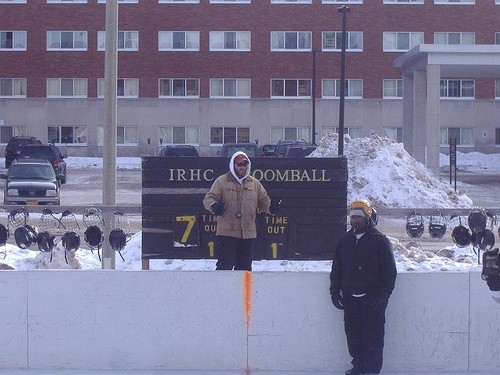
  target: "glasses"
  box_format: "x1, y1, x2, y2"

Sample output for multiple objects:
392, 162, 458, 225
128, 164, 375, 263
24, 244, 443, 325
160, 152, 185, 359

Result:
237, 163, 247, 167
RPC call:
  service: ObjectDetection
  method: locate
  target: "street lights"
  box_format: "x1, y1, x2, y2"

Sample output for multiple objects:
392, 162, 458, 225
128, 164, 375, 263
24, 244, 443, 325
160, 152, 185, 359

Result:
311, 49, 319, 146
337, 5, 350, 155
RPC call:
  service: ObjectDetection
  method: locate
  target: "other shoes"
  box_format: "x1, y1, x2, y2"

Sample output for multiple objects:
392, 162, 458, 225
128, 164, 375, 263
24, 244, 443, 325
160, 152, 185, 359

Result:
345, 369, 352, 375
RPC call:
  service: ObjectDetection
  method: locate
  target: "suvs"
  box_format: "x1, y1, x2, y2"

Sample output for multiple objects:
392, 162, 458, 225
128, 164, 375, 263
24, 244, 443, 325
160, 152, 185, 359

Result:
5, 136, 42, 169
0, 158, 65, 205
159, 138, 317, 158
17, 143, 68, 184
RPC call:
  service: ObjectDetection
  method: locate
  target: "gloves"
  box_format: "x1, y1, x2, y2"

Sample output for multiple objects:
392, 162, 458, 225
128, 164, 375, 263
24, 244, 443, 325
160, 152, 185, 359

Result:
212, 203, 225, 216
261, 211, 276, 217
330, 290, 344, 309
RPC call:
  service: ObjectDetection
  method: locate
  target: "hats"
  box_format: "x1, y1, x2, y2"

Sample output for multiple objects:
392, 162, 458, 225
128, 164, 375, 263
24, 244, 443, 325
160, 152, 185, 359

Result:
235, 155, 248, 162
351, 199, 372, 216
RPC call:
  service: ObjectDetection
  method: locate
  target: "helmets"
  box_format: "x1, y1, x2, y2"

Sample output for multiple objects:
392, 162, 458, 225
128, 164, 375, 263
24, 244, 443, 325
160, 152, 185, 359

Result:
428, 217, 446, 238
468, 211, 494, 250
109, 230, 127, 250
38, 231, 56, 252
452, 225, 471, 248
15, 225, 38, 250
406, 212, 424, 237
84, 225, 102, 246
0, 224, 9, 245
372, 209, 378, 226
61, 232, 80, 253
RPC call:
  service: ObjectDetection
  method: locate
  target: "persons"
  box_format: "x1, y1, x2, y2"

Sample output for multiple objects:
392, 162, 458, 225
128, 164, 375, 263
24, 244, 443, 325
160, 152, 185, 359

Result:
329, 200, 397, 375
203, 151, 273, 271
